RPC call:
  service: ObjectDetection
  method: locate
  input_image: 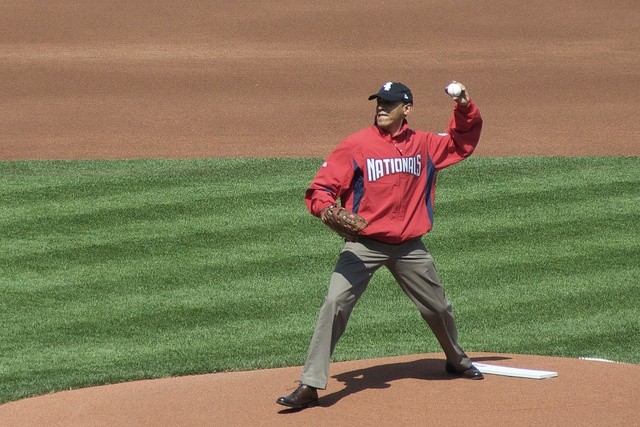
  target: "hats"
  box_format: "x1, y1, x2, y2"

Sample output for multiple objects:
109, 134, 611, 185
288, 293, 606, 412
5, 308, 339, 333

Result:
368, 81, 412, 102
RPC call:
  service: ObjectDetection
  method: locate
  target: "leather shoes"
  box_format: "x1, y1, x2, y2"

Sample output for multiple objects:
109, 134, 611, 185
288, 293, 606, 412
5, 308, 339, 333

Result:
445, 362, 484, 380
276, 384, 319, 408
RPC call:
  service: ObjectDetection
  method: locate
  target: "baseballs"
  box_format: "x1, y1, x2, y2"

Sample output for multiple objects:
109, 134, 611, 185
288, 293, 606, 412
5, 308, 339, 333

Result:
446, 82, 462, 98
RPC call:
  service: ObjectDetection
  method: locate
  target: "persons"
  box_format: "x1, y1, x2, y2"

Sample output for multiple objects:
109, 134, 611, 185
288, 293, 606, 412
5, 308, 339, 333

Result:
274, 79, 484, 409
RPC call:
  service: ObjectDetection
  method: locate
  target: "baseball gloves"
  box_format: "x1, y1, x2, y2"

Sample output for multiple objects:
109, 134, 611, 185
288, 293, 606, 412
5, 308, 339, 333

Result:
320, 203, 369, 242
